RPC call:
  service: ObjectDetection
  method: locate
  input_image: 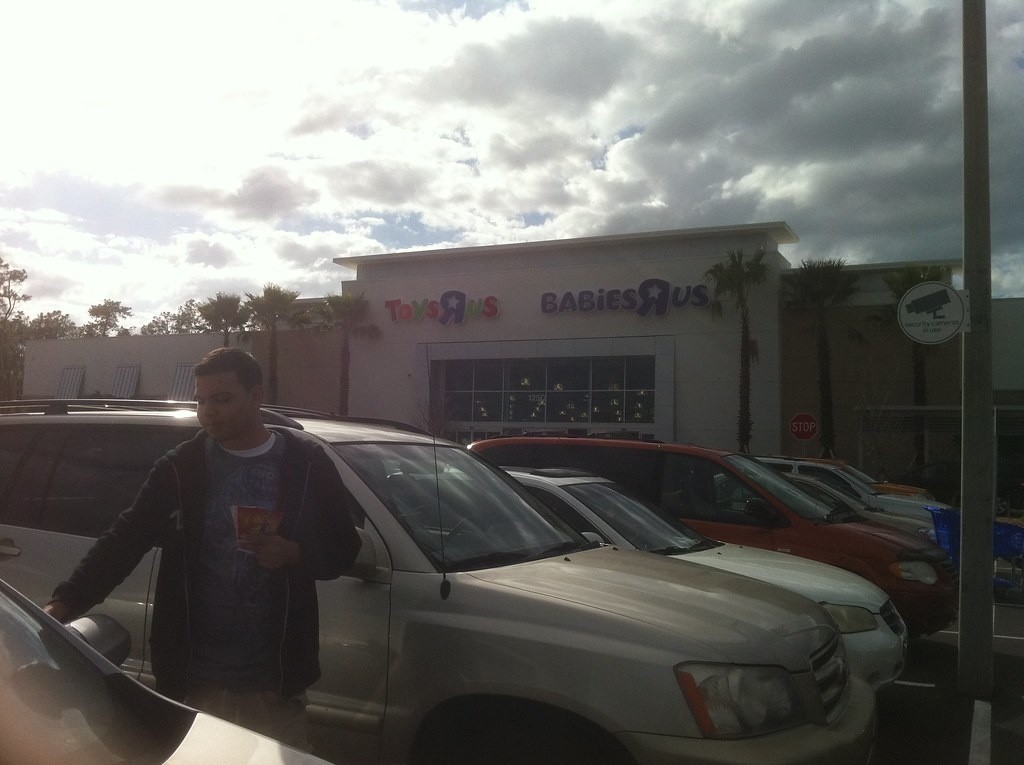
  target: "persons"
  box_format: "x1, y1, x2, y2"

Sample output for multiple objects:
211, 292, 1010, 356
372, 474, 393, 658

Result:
43, 346, 359, 751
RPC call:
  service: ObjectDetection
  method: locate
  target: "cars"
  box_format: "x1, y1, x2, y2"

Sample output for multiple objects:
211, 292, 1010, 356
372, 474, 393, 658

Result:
746, 452, 957, 556
387, 465, 909, 691
0, 581, 329, 764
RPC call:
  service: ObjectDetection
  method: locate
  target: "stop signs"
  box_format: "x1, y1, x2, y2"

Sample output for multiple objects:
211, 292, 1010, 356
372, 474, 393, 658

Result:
790, 413, 819, 441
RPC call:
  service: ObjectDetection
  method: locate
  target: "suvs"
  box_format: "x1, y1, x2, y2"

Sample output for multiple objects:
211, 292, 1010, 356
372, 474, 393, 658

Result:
0, 398, 877, 764
460, 427, 954, 636
